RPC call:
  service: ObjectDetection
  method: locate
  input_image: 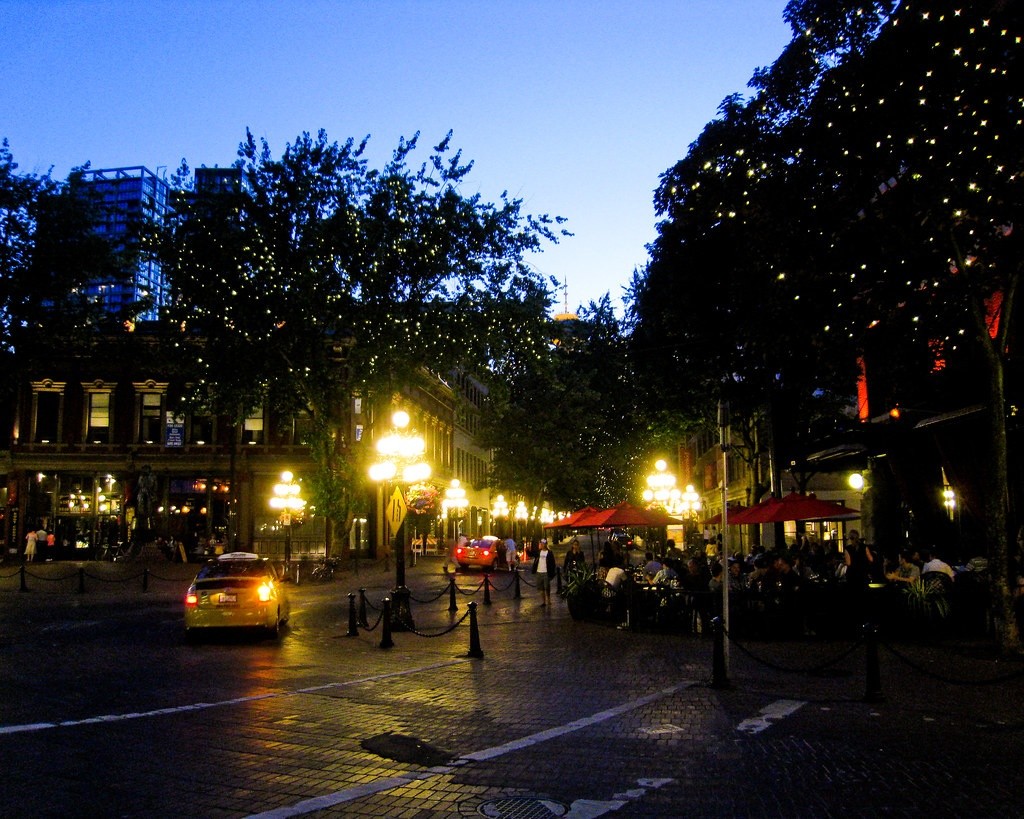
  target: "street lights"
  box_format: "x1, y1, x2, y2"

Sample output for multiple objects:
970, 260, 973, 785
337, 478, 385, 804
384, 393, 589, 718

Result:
442, 480, 468, 545
646, 460, 680, 556
494, 495, 509, 534
514, 501, 528, 540
681, 484, 701, 541
270, 470, 308, 578
368, 412, 430, 630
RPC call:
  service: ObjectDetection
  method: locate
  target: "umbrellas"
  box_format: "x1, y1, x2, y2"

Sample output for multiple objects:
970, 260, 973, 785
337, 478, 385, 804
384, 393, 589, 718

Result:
544, 500, 695, 579
698, 486, 862, 552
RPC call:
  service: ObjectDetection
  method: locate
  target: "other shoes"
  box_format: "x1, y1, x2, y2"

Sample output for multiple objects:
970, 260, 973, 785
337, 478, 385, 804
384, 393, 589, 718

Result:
540, 603, 546, 606
26, 559, 30, 562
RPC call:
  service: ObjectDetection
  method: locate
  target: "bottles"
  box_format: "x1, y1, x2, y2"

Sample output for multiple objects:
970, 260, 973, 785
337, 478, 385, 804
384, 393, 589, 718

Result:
670, 575, 677, 586
639, 563, 645, 571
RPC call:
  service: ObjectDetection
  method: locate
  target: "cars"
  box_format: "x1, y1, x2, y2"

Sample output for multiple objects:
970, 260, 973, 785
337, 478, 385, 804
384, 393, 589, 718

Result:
182, 552, 291, 637
456, 536, 519, 570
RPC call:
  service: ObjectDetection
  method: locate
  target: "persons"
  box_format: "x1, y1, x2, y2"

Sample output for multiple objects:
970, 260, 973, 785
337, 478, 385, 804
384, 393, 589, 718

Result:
562, 526, 988, 637
525, 538, 556, 607
24, 527, 55, 561
136, 463, 159, 517
495, 535, 518, 572
442, 533, 468, 571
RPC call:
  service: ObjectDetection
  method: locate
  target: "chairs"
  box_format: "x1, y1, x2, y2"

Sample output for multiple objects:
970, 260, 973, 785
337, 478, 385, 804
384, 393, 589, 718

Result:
586, 565, 780, 635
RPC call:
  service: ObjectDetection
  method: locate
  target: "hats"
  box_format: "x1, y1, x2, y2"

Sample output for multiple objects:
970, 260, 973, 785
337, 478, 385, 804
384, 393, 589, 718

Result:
539, 539, 547, 543
